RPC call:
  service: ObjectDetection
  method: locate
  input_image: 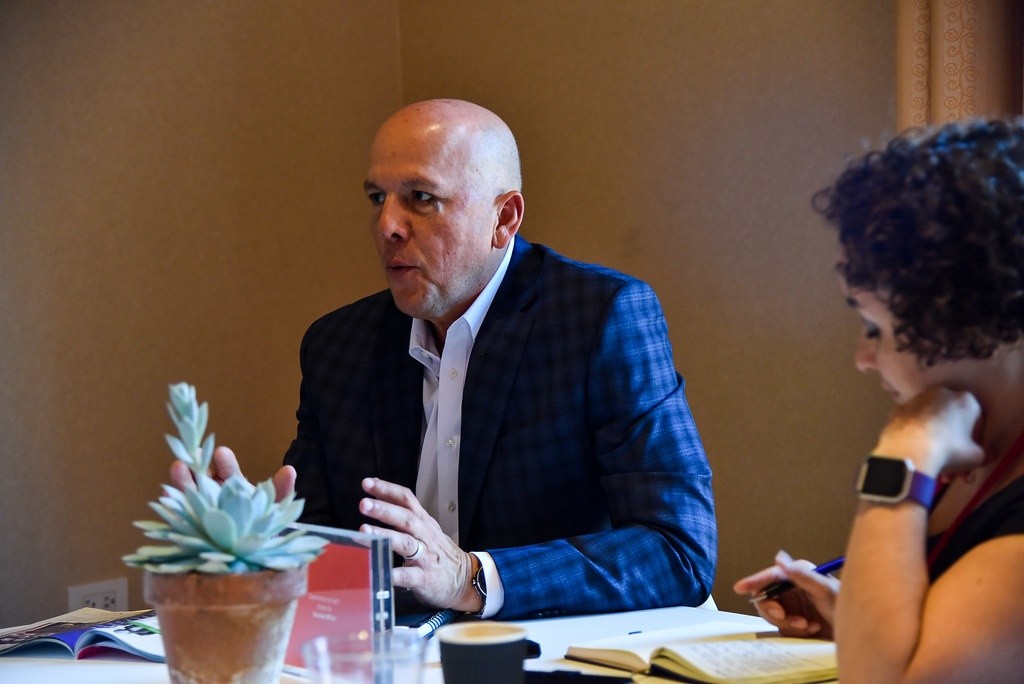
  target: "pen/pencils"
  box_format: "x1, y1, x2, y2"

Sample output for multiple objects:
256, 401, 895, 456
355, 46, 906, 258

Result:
750, 556, 846, 604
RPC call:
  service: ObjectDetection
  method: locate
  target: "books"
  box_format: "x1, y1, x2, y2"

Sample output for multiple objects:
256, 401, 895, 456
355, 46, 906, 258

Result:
564, 610, 837, 684
0, 607, 166, 662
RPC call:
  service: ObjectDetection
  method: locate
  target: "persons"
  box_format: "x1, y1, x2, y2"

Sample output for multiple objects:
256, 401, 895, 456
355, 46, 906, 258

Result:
733, 118, 1024, 684
169, 99, 718, 621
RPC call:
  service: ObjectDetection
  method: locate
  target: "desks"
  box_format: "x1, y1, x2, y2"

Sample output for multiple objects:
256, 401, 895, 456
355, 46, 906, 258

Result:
1, 598, 779, 684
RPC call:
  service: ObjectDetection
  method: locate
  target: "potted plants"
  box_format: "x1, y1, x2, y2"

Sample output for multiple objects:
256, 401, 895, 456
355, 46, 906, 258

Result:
122, 381, 329, 684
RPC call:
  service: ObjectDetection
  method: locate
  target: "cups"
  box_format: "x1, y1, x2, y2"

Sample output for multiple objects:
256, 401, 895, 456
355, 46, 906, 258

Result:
298, 629, 429, 683
437, 621, 527, 684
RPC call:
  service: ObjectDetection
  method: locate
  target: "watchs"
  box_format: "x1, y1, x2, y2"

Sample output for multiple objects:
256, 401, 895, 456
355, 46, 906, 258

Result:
855, 456, 934, 509
472, 557, 487, 613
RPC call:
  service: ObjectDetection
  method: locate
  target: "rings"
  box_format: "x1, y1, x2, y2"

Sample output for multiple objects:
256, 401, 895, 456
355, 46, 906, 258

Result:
403, 540, 423, 560
